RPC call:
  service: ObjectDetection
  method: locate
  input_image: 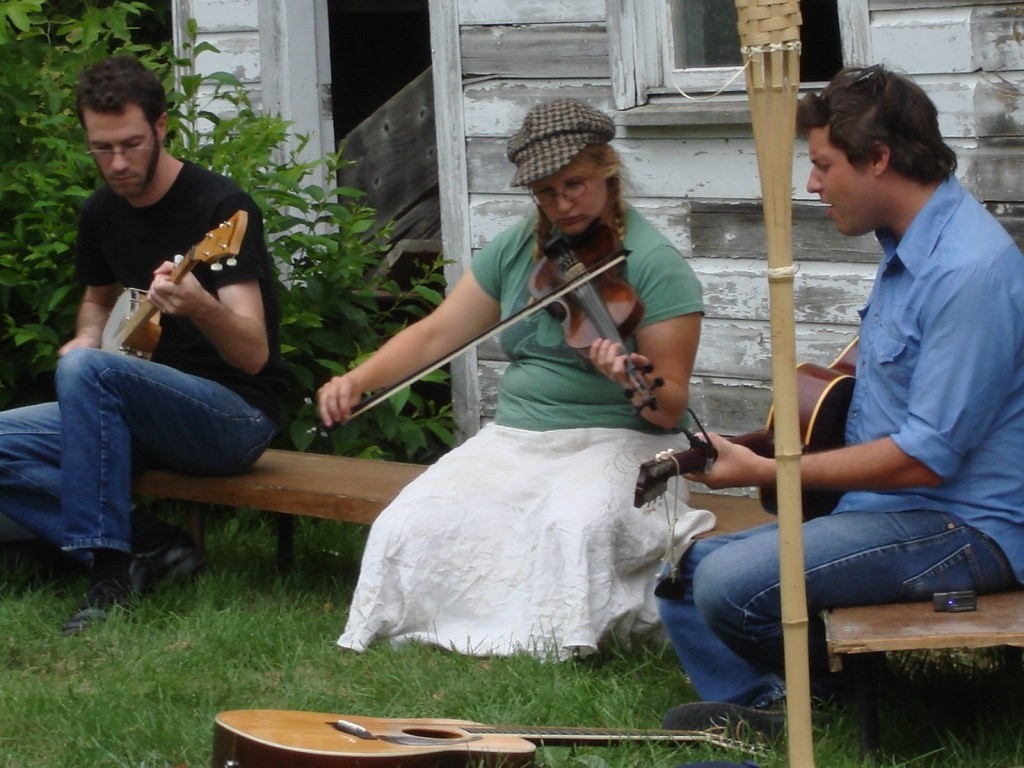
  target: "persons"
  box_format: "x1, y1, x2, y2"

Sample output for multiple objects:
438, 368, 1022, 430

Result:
316, 95, 704, 659
657, 64, 1024, 733
0, 55, 291, 629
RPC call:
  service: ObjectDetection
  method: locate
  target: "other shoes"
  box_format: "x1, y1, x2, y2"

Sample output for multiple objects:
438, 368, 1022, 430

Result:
61, 573, 134, 636
125, 528, 203, 598
660, 699, 835, 745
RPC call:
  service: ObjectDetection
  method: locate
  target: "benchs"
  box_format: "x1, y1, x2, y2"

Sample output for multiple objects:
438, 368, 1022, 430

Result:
129, 448, 1024, 760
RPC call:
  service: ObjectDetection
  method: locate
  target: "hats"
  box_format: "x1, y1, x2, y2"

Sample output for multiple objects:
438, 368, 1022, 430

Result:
505, 98, 616, 188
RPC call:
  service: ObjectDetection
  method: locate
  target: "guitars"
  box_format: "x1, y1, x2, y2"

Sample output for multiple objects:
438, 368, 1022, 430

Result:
99, 210, 248, 363
211, 706, 786, 768
632, 332, 859, 521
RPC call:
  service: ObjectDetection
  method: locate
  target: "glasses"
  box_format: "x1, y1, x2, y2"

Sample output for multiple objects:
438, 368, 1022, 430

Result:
815, 63, 883, 110
85, 143, 150, 162
528, 162, 599, 206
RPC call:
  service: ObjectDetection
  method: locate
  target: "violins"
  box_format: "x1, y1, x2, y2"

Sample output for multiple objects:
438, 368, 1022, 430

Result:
528, 221, 665, 415
